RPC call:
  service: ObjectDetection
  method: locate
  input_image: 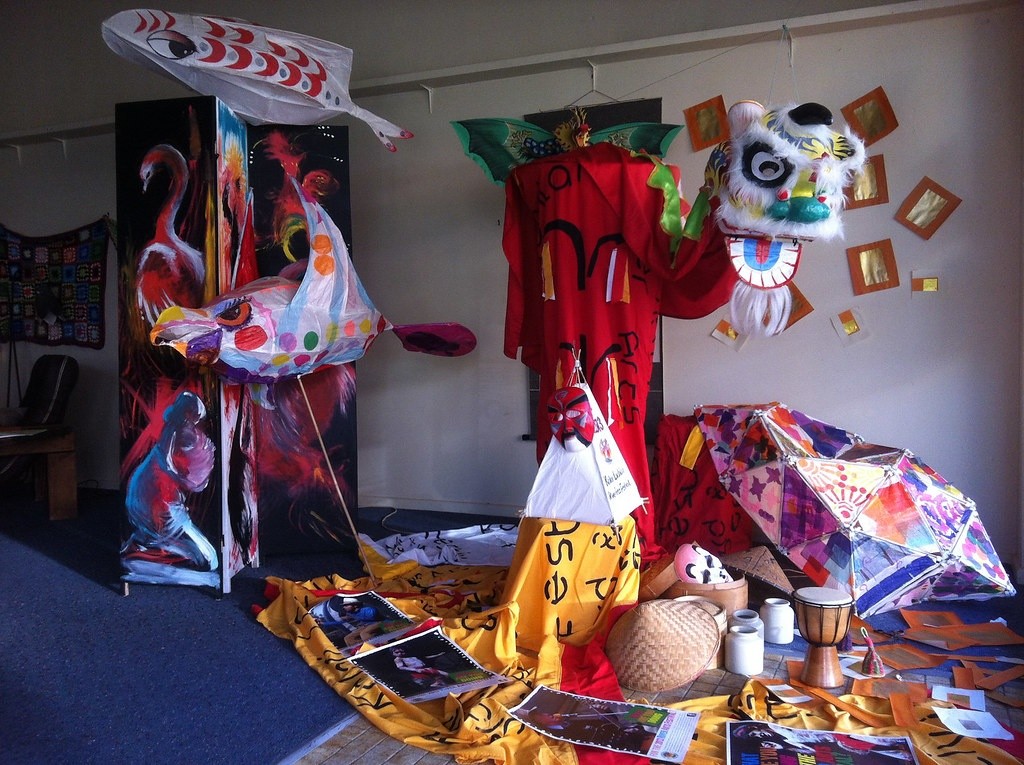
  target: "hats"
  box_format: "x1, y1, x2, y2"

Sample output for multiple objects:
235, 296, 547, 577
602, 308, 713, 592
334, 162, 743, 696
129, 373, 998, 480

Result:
717, 547, 794, 607
342, 598, 359, 605
605, 600, 719, 694
514, 707, 538, 726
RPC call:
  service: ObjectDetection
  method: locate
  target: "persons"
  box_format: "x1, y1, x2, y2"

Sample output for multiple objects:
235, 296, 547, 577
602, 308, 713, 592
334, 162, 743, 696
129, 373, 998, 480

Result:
341, 598, 397, 647
733, 723, 913, 761
516, 704, 659, 755
389, 646, 458, 691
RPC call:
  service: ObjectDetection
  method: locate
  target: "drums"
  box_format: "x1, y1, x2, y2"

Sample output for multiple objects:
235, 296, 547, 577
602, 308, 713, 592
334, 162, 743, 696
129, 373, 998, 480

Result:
791, 587, 855, 688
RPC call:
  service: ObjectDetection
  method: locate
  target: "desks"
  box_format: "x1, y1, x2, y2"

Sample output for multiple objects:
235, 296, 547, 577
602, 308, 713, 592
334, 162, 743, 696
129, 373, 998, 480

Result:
0, 423, 79, 520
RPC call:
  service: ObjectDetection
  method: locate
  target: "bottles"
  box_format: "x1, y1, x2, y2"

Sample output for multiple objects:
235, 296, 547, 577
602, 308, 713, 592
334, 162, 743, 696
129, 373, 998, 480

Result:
728, 609, 765, 639
725, 625, 764, 677
760, 598, 794, 644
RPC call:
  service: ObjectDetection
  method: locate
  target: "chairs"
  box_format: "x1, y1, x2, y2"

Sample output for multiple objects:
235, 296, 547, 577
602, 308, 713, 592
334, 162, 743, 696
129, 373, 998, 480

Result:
17, 355, 80, 425
